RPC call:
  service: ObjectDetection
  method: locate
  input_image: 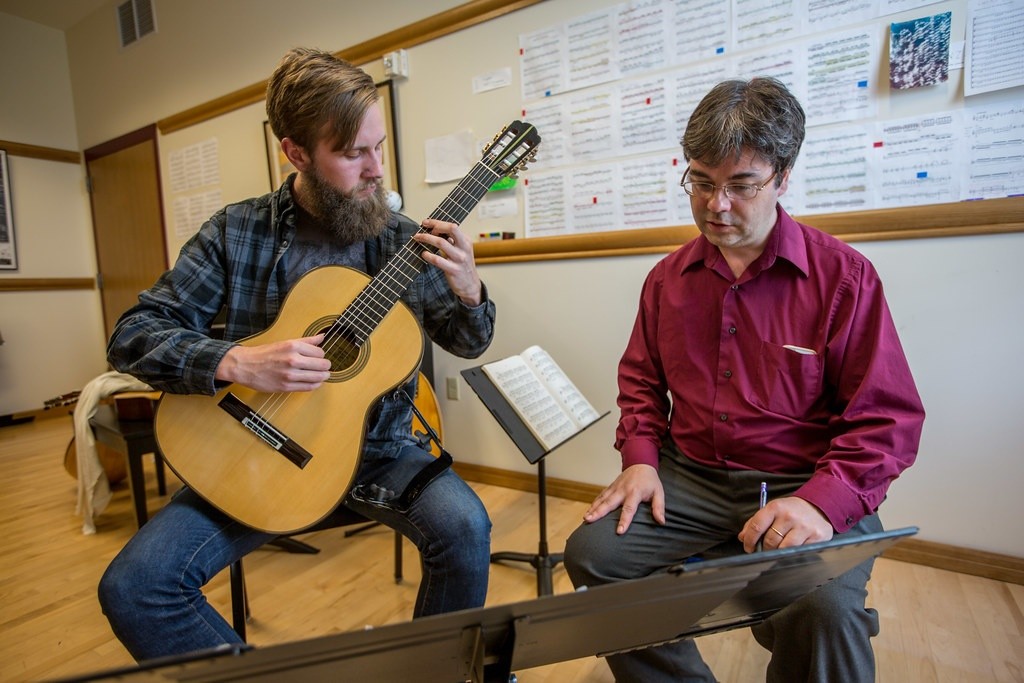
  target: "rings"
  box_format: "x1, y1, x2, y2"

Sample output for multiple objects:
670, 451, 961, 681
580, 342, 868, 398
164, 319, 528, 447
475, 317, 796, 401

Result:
770, 526, 784, 538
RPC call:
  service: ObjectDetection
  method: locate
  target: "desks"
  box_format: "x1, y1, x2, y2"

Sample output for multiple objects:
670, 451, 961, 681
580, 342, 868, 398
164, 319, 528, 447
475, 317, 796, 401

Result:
113, 390, 167, 530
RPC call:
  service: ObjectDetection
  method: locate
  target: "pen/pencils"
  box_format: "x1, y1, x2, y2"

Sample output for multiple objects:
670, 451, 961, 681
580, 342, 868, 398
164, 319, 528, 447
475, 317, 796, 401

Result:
757, 482, 767, 552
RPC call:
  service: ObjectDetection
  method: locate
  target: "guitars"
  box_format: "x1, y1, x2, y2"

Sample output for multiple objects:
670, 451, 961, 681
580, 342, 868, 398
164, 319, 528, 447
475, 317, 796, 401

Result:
151, 120, 542, 537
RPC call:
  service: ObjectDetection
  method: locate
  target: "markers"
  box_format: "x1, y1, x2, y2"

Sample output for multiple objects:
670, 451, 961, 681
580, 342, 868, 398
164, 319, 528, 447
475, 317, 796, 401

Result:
960, 193, 1024, 201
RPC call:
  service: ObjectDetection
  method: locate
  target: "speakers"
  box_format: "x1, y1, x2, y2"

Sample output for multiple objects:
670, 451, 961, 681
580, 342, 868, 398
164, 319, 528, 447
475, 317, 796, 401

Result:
382, 51, 398, 76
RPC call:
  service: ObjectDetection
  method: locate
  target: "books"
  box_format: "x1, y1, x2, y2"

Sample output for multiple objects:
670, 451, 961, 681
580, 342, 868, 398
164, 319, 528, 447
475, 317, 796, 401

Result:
480, 344, 599, 453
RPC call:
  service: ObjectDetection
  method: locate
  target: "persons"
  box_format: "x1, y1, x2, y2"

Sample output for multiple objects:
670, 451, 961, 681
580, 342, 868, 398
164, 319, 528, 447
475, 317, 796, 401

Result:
97, 49, 502, 667
561, 77, 925, 683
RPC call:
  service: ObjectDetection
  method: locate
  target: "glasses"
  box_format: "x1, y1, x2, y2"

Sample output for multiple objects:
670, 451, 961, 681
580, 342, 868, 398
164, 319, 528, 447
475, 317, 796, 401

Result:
680, 163, 787, 200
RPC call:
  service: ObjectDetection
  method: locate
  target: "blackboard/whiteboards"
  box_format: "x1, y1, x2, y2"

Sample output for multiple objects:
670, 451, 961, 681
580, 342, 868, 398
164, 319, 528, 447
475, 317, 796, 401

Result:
150, 2, 1024, 275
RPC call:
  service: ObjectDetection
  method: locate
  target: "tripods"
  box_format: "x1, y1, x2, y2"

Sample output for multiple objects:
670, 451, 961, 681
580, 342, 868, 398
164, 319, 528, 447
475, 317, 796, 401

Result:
459, 345, 611, 597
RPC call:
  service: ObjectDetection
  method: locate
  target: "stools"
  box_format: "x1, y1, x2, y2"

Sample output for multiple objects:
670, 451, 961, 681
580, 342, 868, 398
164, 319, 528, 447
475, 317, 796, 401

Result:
230, 507, 405, 644
